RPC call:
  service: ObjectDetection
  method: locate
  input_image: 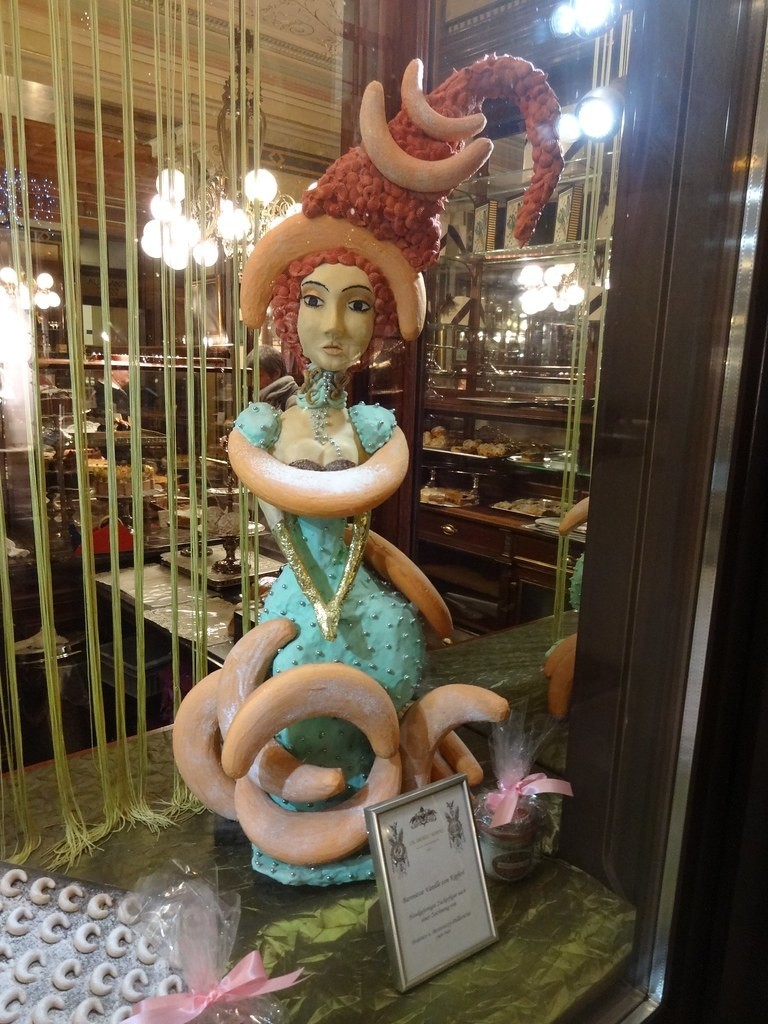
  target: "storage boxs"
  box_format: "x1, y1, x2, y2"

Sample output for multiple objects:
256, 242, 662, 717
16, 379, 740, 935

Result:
504, 191, 530, 246
552, 183, 584, 243
473, 199, 498, 252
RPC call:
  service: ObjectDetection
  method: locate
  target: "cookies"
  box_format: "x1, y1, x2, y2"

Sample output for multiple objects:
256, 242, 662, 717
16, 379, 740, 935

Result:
0, 870, 189, 1024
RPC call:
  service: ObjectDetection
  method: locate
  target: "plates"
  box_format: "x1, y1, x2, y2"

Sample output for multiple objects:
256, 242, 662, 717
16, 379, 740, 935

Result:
507, 456, 551, 464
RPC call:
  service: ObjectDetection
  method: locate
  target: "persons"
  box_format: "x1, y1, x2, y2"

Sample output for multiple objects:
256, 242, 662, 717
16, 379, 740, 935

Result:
172, 53, 565, 888
92, 353, 131, 426
241, 342, 297, 407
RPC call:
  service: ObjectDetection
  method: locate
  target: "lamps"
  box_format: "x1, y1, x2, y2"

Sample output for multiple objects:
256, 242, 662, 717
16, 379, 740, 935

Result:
0, 0, 303, 310
577, 75, 626, 141
568, 0, 633, 40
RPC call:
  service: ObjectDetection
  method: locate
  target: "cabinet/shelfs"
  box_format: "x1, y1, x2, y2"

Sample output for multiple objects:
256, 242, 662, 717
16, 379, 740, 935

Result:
411, 0, 634, 630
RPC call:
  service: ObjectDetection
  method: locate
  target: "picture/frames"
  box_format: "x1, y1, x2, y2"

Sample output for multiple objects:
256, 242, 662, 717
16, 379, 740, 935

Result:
363, 769, 501, 994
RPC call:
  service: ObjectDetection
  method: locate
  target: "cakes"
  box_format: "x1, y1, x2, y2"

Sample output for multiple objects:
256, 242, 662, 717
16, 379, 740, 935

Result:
93, 465, 155, 494
173, 50, 566, 886
